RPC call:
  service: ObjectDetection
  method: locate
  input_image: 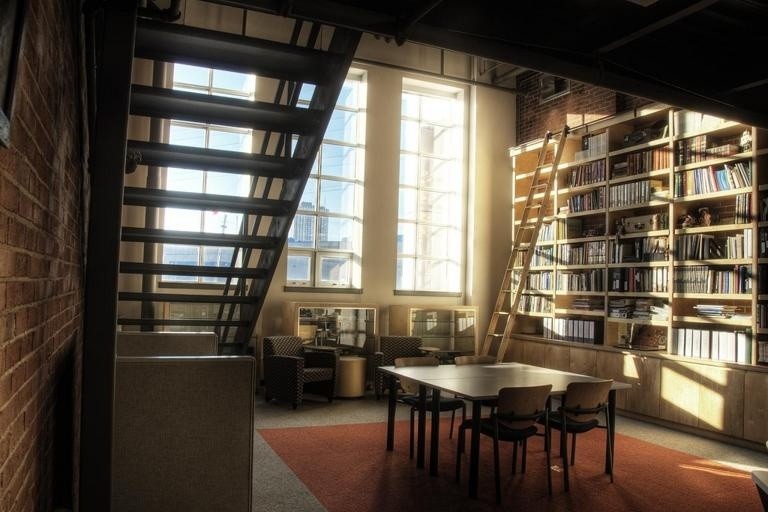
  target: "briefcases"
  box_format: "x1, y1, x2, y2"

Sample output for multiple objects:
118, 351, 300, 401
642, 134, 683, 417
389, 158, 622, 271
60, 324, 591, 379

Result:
620, 213, 665, 234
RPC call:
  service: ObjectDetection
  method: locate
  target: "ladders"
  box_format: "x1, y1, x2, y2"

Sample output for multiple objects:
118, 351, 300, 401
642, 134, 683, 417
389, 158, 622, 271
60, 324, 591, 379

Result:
480, 125, 570, 363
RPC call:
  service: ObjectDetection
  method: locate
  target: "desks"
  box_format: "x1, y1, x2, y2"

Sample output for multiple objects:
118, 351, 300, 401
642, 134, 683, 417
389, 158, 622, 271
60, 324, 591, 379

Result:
377, 363, 632, 500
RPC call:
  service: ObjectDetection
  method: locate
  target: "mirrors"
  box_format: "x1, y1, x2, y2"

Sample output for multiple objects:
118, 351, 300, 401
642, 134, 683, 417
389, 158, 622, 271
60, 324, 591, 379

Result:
297, 306, 376, 395
410, 307, 478, 366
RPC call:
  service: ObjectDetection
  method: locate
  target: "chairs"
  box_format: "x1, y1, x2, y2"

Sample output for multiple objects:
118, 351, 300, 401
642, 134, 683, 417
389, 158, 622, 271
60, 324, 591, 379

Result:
395, 352, 613, 503
262, 336, 340, 411
372, 333, 430, 401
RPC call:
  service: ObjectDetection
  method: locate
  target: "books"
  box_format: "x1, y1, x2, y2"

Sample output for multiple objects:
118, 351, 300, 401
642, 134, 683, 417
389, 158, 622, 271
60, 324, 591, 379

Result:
608, 227, 768, 366
560, 132, 607, 214
512, 218, 607, 341
609, 120, 669, 207
735, 193, 751, 224
672, 129, 753, 197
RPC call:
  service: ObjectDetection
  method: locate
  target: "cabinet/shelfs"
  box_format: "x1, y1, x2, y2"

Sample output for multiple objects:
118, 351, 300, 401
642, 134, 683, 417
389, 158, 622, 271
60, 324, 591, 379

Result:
495, 99, 768, 452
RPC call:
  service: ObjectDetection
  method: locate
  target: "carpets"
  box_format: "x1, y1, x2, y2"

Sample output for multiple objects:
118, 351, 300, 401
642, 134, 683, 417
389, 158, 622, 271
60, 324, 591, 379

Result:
255, 413, 762, 512
